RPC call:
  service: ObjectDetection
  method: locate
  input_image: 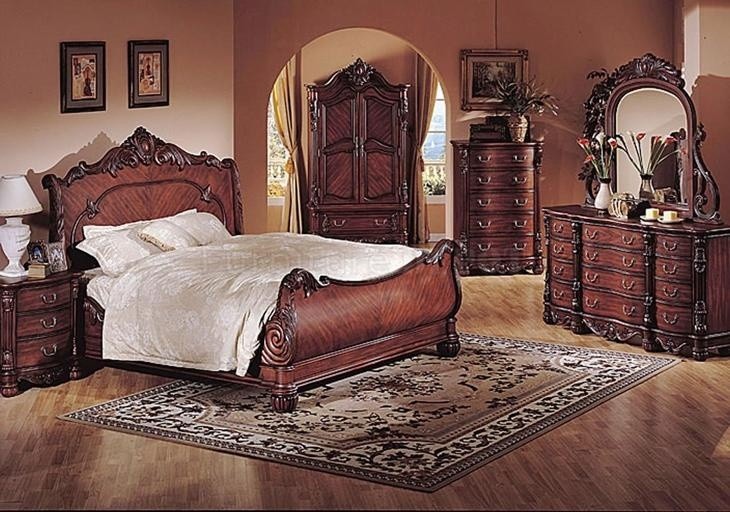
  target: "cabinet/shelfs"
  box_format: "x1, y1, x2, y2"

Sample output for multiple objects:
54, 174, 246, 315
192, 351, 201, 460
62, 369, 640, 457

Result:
306, 56, 409, 247
541, 202, 729, 361
450, 136, 546, 277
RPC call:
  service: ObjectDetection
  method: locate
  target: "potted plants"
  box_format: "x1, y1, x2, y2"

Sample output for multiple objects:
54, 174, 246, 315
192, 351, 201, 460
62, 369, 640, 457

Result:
491, 77, 561, 143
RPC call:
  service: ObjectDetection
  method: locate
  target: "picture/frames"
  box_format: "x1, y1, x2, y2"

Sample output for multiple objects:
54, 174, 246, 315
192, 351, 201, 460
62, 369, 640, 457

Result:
459, 46, 528, 110
28, 242, 43, 264
58, 40, 107, 113
126, 39, 171, 109
45, 241, 67, 274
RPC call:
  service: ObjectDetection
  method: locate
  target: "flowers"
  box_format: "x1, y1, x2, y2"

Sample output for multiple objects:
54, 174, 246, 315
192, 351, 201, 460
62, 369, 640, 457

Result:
576, 132, 621, 180
614, 131, 681, 174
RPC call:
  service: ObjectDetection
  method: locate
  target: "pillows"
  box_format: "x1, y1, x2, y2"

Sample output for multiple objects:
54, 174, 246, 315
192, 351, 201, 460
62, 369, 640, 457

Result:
178, 211, 232, 244
139, 219, 197, 251
76, 226, 158, 277
82, 222, 120, 237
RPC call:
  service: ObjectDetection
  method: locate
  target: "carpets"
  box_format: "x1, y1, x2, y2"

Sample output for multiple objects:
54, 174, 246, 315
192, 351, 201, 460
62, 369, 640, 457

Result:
56, 331, 683, 494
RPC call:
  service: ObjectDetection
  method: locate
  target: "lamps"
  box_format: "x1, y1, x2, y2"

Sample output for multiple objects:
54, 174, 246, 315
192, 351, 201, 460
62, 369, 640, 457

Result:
0, 174, 44, 281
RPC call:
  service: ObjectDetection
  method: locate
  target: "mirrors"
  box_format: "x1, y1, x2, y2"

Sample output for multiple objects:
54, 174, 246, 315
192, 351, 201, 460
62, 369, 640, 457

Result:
606, 78, 689, 211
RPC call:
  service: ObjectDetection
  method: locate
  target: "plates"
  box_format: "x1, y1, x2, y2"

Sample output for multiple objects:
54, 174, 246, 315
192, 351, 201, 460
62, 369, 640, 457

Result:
639, 216, 659, 220
657, 219, 684, 223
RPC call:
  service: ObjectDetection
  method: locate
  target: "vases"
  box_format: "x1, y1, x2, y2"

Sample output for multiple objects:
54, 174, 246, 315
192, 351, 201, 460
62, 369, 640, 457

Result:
592, 181, 616, 209
640, 175, 655, 206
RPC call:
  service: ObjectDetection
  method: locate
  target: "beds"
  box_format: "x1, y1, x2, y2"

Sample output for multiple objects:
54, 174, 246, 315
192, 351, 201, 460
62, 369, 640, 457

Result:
41, 123, 464, 415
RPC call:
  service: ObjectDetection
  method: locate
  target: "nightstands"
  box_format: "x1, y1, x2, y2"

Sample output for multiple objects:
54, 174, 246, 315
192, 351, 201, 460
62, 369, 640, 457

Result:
1, 265, 83, 398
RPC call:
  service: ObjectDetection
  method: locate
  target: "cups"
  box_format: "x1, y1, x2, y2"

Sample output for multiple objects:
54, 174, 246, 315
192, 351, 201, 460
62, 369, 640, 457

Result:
645, 208, 659, 218
663, 211, 677, 220
